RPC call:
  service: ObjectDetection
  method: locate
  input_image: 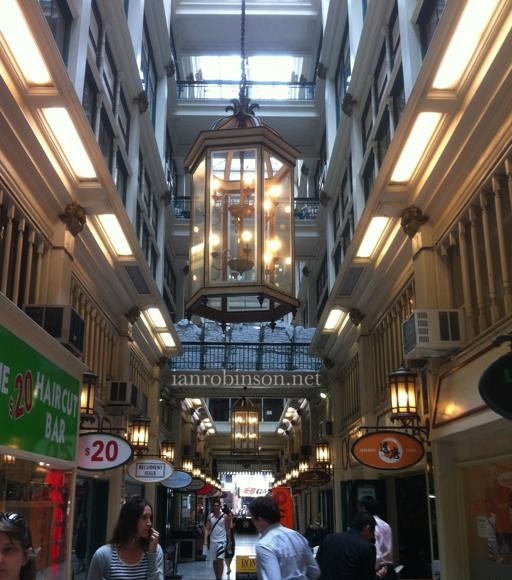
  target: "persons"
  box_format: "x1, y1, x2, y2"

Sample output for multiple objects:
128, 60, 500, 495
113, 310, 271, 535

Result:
0, 511, 38, 580
87, 496, 165, 580
191, 496, 400, 580
74, 509, 88, 575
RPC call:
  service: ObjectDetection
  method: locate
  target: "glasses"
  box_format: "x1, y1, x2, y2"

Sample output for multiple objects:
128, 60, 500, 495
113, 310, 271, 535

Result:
1, 512, 25, 526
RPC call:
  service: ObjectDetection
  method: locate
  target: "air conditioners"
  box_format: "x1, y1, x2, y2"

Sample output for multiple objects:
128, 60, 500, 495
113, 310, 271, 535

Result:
108, 379, 139, 409
398, 307, 468, 364
22, 301, 89, 358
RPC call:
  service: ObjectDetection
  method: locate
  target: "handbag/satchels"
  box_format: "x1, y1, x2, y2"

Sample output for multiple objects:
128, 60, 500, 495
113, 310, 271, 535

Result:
207, 534, 210, 550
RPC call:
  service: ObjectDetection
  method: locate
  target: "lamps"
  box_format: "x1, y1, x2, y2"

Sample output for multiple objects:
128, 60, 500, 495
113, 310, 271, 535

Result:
184, 1, 308, 331
312, 434, 331, 467
130, 412, 151, 451
384, 361, 422, 423
80, 368, 99, 423
160, 438, 201, 478
227, 396, 261, 458
